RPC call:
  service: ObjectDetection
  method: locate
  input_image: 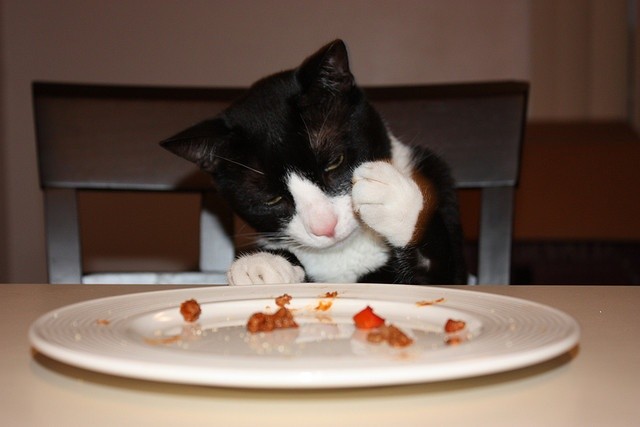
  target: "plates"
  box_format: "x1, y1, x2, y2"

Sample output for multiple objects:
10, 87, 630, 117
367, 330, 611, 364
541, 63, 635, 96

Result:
25, 279, 584, 392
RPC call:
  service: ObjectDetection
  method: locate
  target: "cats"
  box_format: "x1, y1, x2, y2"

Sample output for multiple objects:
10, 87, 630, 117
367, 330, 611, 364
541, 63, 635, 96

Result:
159, 38, 469, 285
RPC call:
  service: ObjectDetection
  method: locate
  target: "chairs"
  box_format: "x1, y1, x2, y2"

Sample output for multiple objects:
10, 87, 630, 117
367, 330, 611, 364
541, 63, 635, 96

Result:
32, 81, 530, 286
